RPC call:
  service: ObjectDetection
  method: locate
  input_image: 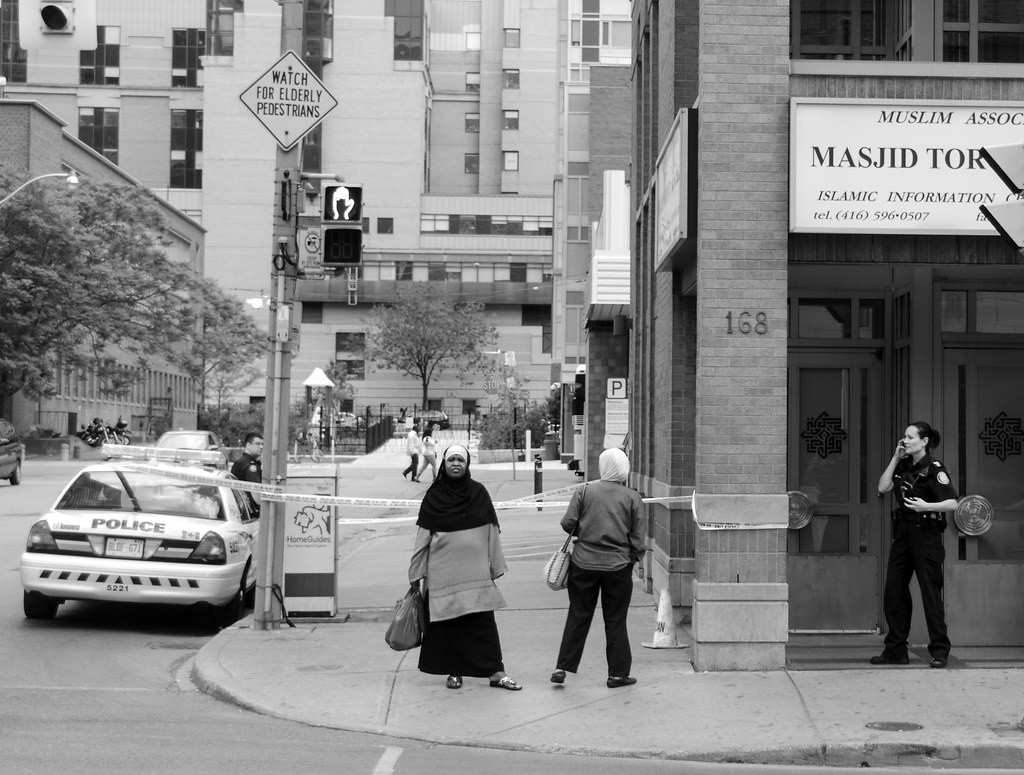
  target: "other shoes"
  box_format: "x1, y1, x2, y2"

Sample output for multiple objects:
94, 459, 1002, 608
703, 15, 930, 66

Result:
869, 655, 910, 664
928, 654, 948, 668
402, 472, 407, 479
410, 477, 421, 482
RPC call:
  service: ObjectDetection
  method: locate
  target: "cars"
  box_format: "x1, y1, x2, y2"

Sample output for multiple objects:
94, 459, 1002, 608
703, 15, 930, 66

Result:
151, 428, 231, 471
20, 445, 261, 631
334, 410, 361, 427
393, 409, 451, 431
0, 416, 24, 484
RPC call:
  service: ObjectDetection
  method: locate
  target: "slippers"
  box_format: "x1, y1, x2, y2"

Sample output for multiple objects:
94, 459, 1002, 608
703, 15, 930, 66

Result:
445, 674, 463, 689
489, 676, 522, 690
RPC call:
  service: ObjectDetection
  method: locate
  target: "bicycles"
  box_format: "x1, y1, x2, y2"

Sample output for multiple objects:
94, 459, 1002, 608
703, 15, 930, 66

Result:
309, 436, 323, 463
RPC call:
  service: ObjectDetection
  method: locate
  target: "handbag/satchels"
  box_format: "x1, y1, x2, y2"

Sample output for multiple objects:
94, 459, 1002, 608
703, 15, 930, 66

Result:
381, 580, 427, 652
543, 547, 574, 592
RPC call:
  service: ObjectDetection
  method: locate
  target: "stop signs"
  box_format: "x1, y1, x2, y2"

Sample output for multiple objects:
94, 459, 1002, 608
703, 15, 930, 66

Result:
482, 373, 502, 394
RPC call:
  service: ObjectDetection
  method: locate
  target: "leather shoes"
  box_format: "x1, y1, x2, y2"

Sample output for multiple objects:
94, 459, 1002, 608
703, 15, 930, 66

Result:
606, 675, 638, 688
550, 670, 567, 684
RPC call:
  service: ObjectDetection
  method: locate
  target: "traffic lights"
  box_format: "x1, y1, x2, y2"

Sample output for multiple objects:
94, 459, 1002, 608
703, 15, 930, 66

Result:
320, 227, 366, 269
319, 185, 364, 223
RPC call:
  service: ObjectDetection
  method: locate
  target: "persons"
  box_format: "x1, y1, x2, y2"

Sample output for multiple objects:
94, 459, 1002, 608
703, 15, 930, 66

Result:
231, 432, 264, 523
870, 421, 960, 668
414, 428, 437, 482
550, 447, 648, 689
408, 444, 522, 691
402, 425, 422, 483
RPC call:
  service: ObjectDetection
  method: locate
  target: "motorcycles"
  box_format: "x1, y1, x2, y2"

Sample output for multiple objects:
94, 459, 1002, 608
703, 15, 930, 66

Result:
75, 416, 131, 448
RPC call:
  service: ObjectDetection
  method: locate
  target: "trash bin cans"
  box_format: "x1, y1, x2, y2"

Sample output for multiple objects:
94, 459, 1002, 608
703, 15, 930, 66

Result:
544, 432, 559, 460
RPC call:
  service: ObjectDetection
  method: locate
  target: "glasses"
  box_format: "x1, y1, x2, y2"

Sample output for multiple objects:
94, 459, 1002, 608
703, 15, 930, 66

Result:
250, 442, 264, 446
